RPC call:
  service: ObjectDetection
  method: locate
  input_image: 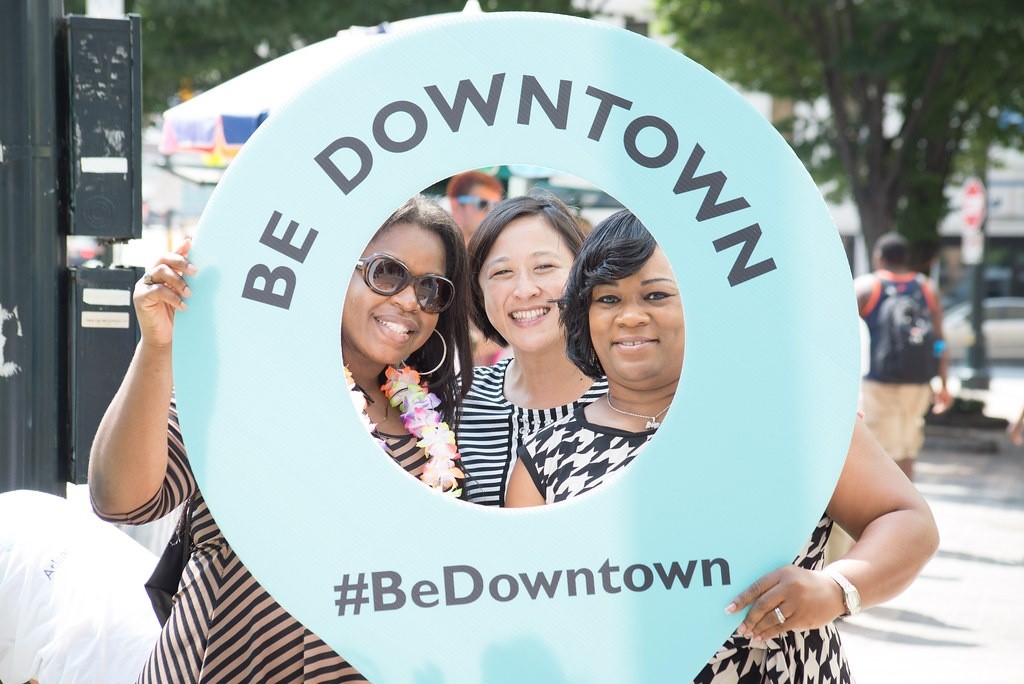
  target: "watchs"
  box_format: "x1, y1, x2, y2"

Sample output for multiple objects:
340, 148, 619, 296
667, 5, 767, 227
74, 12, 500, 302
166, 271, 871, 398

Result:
823, 570, 861, 618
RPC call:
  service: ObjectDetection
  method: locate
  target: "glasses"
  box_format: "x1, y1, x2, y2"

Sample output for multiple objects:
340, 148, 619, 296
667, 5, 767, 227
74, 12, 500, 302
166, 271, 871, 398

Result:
355, 253, 456, 314
460, 197, 497, 211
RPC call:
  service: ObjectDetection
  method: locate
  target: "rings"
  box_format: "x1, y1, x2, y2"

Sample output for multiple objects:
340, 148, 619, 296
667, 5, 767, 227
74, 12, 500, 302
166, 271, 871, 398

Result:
774, 606, 786, 624
144, 271, 154, 284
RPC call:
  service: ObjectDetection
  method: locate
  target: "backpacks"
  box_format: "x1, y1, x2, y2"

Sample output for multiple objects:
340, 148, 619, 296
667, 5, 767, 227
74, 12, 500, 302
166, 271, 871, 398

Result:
864, 271, 947, 383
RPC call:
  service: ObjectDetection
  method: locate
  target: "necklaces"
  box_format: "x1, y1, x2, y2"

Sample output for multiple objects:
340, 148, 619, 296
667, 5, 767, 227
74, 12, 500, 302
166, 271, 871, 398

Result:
343, 363, 466, 492
367, 406, 390, 426
606, 390, 672, 429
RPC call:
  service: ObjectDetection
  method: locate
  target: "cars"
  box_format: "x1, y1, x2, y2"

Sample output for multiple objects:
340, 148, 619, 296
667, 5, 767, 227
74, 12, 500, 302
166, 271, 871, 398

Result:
937, 294, 1024, 361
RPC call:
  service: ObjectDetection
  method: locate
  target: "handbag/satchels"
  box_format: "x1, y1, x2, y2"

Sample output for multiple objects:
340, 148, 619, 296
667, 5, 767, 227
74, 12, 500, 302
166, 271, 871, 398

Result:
144, 492, 193, 627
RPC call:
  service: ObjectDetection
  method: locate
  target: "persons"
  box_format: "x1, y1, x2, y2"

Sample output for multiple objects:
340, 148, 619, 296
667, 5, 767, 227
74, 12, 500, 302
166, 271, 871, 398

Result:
447, 171, 514, 366
574, 216, 593, 238
450, 196, 609, 508
88, 193, 474, 684
1006, 406, 1024, 446
854, 232, 953, 482
505, 208, 941, 684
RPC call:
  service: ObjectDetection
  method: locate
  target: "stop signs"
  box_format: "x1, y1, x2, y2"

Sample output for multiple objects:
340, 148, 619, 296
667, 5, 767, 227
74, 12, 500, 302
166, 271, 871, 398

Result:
962, 178, 987, 230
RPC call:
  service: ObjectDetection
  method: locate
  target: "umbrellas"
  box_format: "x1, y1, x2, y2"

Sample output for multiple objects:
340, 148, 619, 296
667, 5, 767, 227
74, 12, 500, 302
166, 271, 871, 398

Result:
159, 0, 488, 184
